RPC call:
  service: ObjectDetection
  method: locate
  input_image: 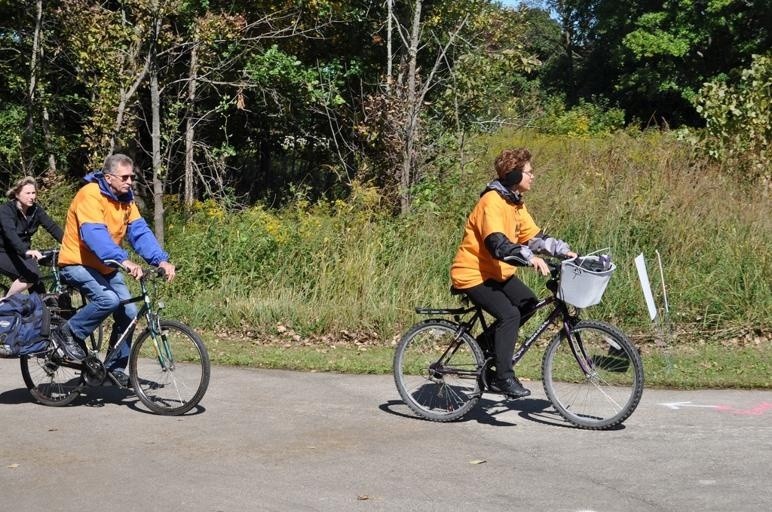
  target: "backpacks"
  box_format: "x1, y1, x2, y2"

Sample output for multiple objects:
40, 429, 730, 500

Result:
0, 293, 50, 357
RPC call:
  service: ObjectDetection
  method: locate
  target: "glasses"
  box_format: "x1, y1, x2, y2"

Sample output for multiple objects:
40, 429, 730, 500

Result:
111, 172, 138, 183
526, 168, 537, 177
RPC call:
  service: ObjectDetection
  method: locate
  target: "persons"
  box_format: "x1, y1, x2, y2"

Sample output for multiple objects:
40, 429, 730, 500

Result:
450, 148, 578, 398
0, 176, 63, 301
51, 154, 176, 386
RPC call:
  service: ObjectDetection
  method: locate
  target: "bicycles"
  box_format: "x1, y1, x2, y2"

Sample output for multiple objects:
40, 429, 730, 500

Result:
0, 248, 211, 416
393, 254, 645, 430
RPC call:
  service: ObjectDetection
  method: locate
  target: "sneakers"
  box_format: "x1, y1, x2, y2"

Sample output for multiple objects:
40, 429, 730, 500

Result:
113, 369, 134, 388
51, 323, 87, 362
491, 375, 531, 397
